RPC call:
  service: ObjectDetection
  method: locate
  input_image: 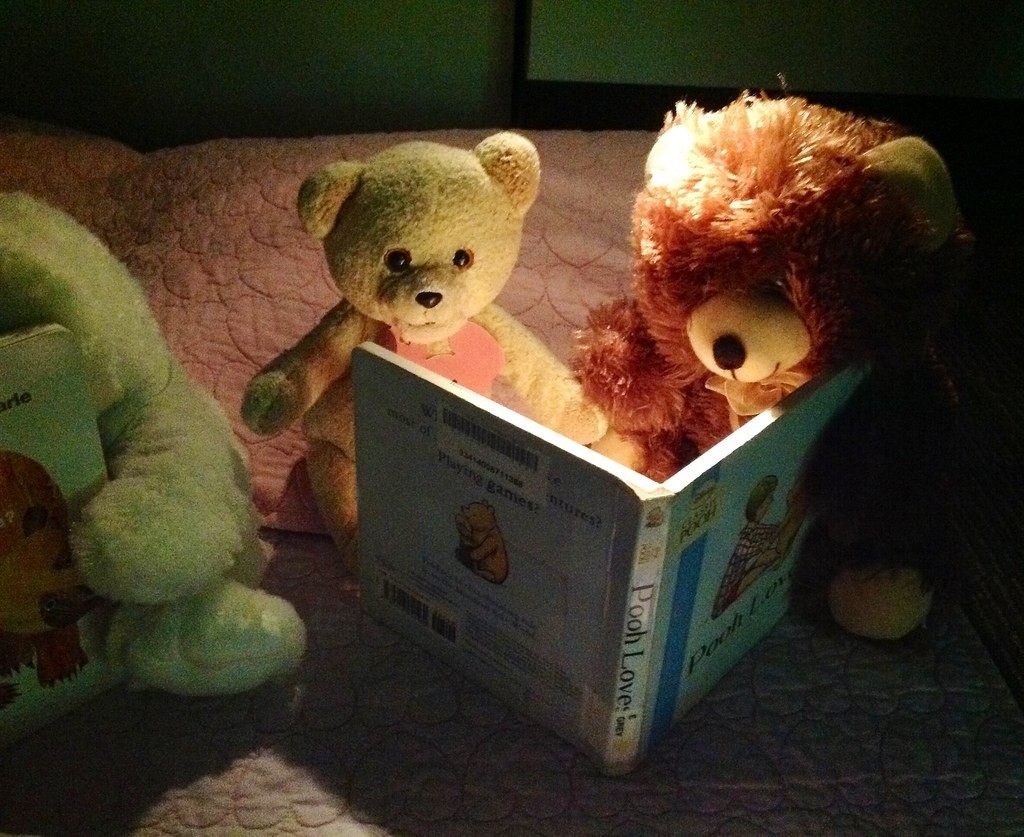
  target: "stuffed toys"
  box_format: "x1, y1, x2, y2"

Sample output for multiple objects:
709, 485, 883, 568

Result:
1, 192, 311, 700
572, 88, 978, 643
240, 127, 608, 576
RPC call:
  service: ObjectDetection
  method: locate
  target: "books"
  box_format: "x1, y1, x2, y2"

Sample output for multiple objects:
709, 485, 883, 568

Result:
350, 342, 873, 775
0, 323, 122, 742
